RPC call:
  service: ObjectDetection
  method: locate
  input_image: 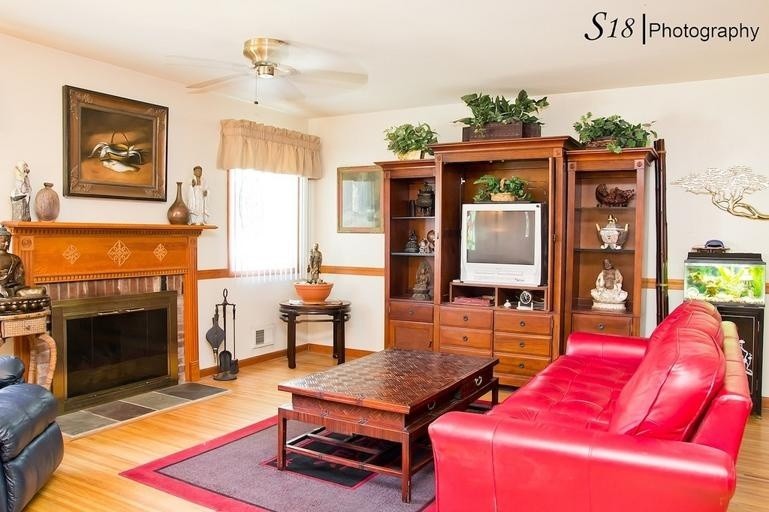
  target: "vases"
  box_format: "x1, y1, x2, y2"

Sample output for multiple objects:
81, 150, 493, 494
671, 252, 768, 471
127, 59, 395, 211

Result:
167, 179, 191, 225
32, 182, 61, 223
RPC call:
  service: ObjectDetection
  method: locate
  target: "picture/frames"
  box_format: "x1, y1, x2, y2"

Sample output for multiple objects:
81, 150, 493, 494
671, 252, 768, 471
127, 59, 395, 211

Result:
62, 84, 171, 203
334, 164, 386, 235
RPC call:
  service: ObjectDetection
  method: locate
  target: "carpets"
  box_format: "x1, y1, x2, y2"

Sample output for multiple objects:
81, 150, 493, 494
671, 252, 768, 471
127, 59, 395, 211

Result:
116, 398, 503, 512
51, 378, 232, 439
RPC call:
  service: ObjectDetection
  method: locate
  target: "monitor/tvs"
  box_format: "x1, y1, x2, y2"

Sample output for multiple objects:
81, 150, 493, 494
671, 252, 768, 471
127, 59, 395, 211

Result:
461, 203, 548, 287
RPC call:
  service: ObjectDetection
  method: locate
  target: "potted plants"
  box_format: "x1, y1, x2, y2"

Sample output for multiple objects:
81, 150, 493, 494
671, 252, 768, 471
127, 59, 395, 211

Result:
572, 111, 659, 156
451, 88, 551, 142
469, 174, 538, 203
379, 123, 438, 161
293, 242, 336, 305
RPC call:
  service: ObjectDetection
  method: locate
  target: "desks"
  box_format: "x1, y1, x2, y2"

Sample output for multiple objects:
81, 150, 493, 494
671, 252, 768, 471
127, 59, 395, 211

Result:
278, 297, 352, 369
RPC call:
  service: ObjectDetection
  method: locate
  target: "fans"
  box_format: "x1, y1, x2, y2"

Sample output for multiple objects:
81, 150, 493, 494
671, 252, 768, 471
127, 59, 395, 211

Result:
182, 39, 369, 124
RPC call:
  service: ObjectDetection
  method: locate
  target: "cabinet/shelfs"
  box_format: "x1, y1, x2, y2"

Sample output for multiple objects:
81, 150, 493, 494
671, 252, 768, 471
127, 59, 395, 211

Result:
375, 136, 661, 389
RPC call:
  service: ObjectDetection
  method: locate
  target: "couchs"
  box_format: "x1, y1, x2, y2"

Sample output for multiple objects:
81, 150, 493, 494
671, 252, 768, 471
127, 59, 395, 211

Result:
0, 354, 64, 512
427, 296, 753, 511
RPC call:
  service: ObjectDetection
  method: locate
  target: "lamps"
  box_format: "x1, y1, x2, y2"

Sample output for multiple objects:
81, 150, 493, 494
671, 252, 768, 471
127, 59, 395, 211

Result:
232, 67, 290, 108
